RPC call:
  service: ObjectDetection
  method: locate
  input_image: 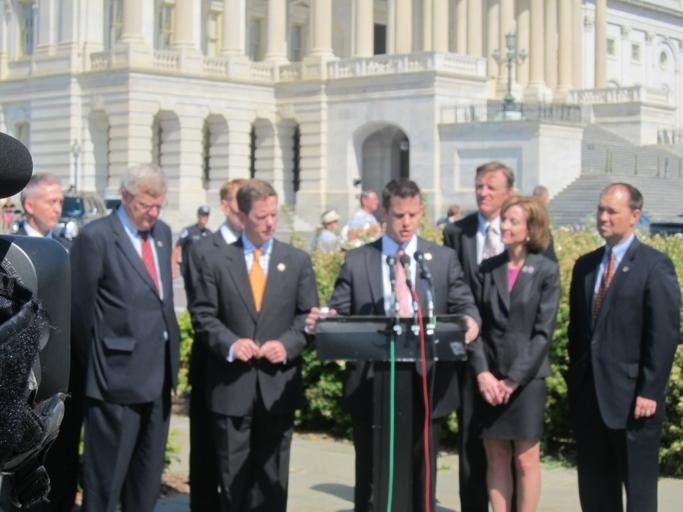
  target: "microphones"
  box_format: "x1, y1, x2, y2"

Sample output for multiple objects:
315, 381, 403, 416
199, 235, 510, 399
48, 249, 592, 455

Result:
400, 254, 410, 286
386, 255, 397, 285
414, 251, 431, 286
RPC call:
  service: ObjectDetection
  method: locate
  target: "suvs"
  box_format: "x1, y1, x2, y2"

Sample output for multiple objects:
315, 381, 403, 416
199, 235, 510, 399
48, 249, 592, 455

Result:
10, 191, 107, 240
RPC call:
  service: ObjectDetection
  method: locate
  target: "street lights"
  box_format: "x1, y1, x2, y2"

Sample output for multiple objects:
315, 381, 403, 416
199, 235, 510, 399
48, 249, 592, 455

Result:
71, 140, 80, 190
492, 35, 526, 120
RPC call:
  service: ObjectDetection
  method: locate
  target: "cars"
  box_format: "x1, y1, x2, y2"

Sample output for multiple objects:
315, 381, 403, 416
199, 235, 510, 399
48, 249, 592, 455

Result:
101, 193, 122, 215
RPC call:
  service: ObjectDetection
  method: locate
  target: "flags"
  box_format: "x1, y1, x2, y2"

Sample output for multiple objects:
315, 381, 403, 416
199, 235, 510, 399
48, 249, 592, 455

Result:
186, 176, 321, 511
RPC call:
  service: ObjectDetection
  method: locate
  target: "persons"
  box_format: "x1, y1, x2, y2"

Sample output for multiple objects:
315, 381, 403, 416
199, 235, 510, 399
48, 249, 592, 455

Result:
67, 163, 183, 510
565, 181, 681, 511
443, 159, 559, 511
0, 159, 552, 286
303, 177, 483, 511
469, 192, 561, 512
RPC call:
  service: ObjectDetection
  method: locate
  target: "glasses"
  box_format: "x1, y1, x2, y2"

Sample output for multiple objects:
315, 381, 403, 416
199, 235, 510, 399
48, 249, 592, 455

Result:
135, 200, 161, 208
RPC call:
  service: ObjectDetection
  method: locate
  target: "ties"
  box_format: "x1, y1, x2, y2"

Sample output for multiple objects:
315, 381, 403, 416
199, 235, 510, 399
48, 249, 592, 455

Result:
484, 225, 496, 258
249, 249, 266, 311
593, 253, 614, 321
138, 232, 159, 294
394, 249, 413, 318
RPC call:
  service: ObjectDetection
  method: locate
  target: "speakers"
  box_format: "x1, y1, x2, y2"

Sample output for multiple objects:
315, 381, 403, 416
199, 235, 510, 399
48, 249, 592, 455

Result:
1, 234, 71, 406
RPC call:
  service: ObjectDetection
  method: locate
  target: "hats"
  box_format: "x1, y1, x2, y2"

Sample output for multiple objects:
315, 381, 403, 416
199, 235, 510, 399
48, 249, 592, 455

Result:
321, 211, 339, 224
199, 207, 208, 214
